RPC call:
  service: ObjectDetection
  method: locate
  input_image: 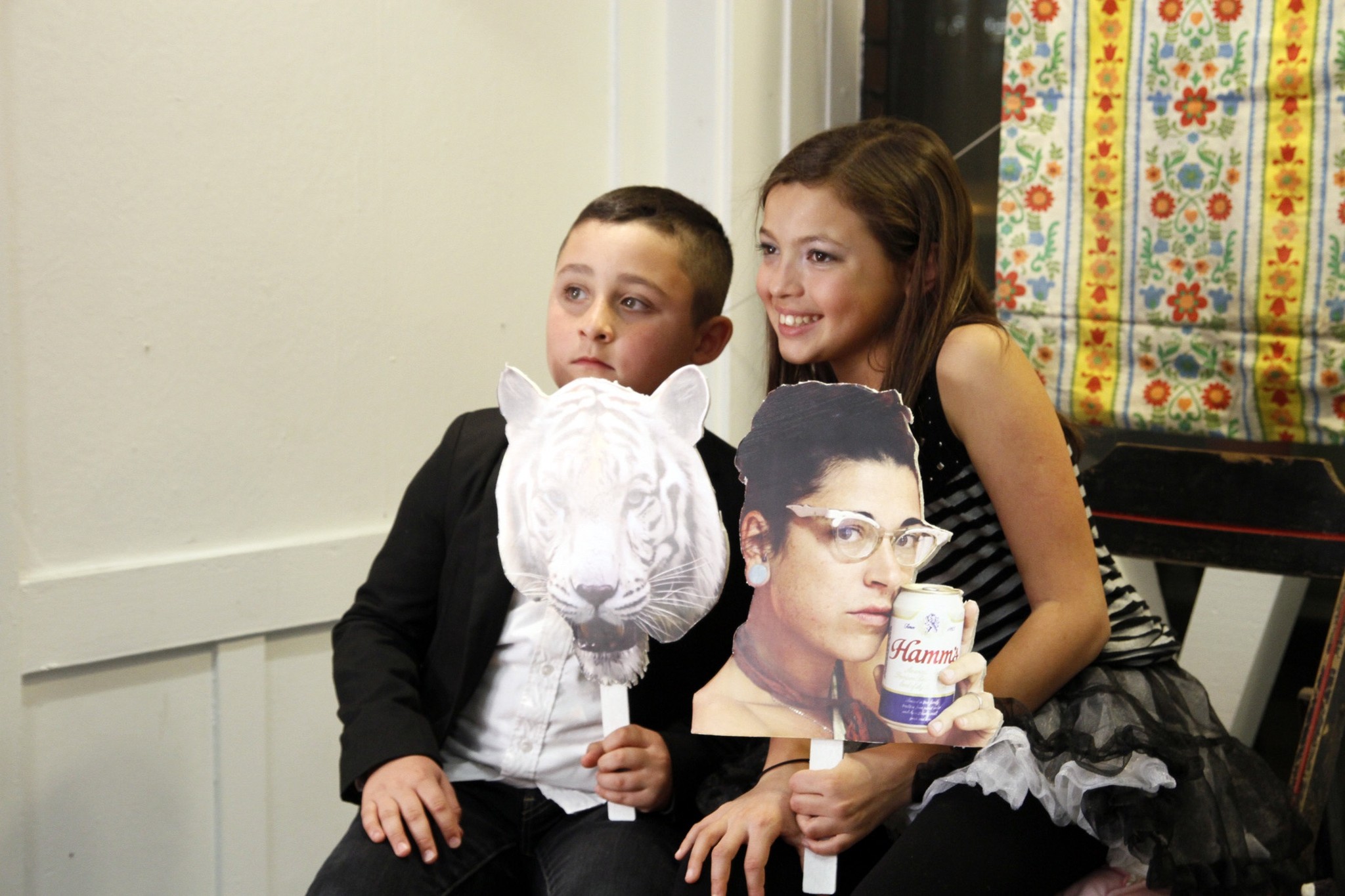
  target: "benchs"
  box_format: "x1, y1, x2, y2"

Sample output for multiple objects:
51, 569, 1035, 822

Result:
1052, 430, 1345, 896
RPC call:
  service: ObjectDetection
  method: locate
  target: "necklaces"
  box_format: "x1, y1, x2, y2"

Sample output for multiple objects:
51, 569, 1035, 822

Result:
774, 697, 834, 734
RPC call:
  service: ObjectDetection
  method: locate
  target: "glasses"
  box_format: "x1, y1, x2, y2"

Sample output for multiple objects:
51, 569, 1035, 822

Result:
784, 503, 954, 569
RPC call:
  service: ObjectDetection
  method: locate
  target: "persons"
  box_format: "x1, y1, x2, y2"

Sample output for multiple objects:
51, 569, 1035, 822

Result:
306, 186, 753, 896
691, 382, 1003, 747
674, 117, 1332, 896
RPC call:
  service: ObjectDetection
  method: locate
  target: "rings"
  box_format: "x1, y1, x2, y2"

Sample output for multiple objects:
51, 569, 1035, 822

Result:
965, 692, 982, 710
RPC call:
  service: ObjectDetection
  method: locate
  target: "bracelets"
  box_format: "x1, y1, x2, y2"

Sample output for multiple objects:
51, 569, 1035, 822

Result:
758, 758, 810, 777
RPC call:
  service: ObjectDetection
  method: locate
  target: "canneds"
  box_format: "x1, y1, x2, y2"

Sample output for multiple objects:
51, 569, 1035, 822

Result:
879, 584, 964, 734
